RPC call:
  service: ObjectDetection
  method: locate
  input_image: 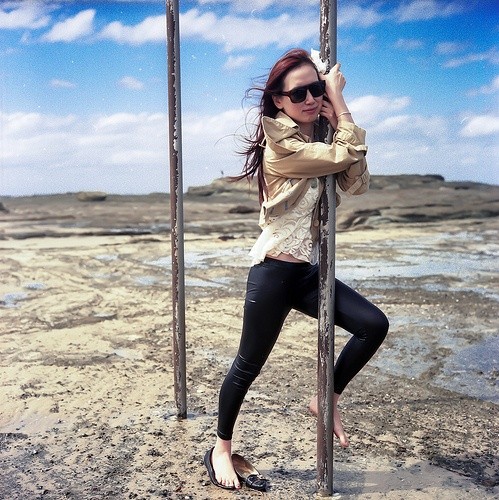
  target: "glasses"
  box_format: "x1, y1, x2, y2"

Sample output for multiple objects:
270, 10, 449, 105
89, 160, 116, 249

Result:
276, 80, 326, 103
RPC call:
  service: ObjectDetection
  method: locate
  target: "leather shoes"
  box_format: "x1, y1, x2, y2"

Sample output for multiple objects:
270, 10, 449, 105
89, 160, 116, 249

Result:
231, 454, 267, 490
204, 446, 234, 489
